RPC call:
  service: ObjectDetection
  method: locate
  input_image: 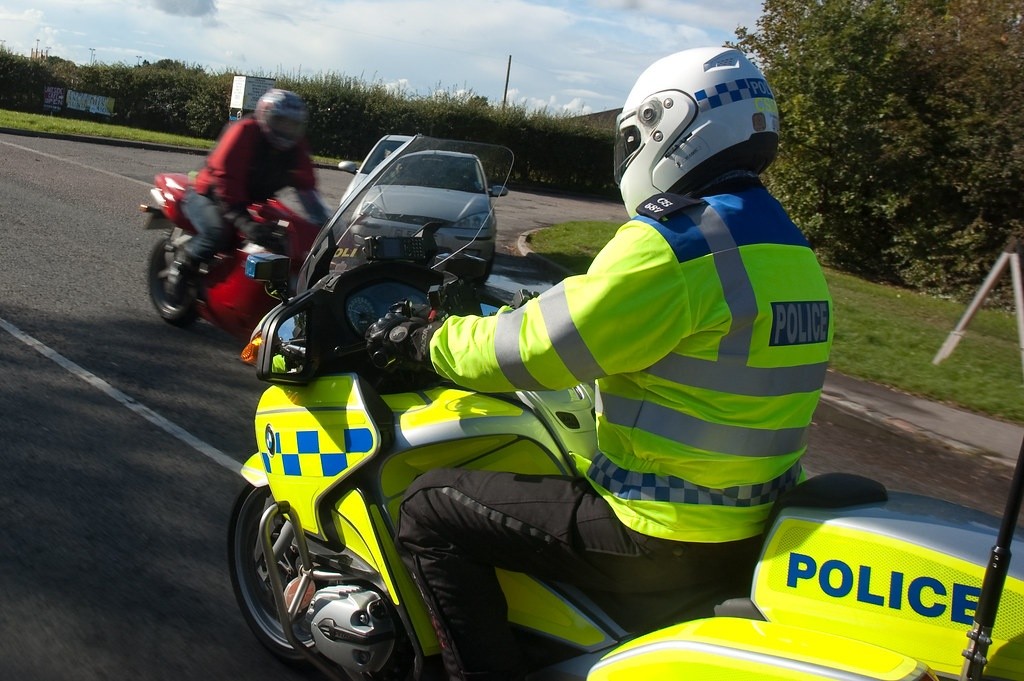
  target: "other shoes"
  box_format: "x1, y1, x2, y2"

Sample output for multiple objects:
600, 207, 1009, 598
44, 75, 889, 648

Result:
171, 248, 196, 283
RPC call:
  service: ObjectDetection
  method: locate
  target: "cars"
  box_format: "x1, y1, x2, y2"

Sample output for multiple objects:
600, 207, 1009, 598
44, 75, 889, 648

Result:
335, 131, 415, 207
353, 150, 510, 287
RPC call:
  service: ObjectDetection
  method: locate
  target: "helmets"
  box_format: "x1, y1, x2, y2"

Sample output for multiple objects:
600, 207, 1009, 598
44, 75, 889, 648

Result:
611, 47, 781, 217
257, 89, 309, 150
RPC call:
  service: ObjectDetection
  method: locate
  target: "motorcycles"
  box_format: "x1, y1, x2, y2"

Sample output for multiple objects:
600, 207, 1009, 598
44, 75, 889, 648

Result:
224, 216, 1023, 681
135, 165, 355, 333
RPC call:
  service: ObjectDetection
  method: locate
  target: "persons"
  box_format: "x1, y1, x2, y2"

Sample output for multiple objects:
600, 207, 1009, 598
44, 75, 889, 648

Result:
163, 87, 330, 306
366, 47, 835, 681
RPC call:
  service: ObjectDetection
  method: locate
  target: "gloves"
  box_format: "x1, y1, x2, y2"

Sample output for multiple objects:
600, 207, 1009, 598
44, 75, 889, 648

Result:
365, 309, 443, 372
234, 213, 276, 247
